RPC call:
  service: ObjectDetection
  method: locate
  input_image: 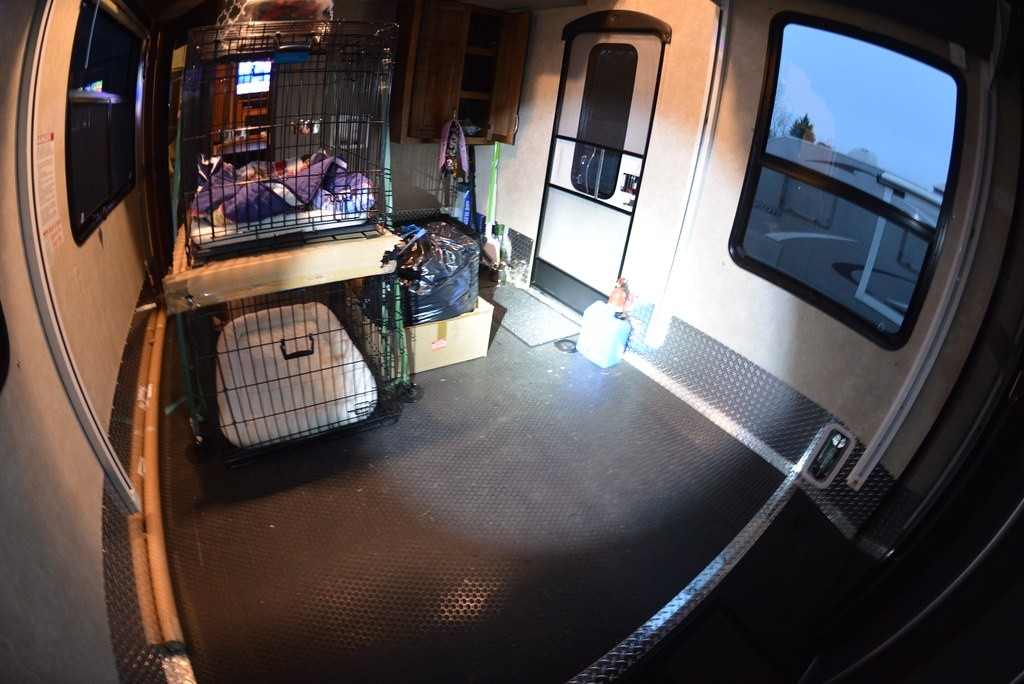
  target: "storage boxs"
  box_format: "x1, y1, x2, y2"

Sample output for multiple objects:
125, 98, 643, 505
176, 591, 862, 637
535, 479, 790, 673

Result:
350, 291, 494, 382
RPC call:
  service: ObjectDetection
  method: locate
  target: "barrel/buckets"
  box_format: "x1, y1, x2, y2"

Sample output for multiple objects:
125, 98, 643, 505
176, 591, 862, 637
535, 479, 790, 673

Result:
576, 300, 631, 369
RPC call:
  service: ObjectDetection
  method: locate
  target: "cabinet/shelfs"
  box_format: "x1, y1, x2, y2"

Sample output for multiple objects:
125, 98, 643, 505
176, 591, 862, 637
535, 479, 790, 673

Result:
378, 0, 533, 146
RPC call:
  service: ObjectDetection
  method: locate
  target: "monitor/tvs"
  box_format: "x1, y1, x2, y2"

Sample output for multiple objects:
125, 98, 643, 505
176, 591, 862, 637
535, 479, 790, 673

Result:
235, 61, 272, 96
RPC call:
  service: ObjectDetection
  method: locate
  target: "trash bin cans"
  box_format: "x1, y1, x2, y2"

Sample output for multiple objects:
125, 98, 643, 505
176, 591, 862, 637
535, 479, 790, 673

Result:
576, 301, 632, 370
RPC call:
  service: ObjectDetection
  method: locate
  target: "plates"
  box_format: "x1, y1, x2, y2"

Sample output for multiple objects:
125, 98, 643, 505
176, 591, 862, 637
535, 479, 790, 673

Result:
452, 123, 481, 136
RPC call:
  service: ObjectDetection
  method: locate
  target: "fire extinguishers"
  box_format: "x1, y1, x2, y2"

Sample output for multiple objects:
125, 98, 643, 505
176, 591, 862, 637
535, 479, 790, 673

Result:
607, 278, 628, 309
